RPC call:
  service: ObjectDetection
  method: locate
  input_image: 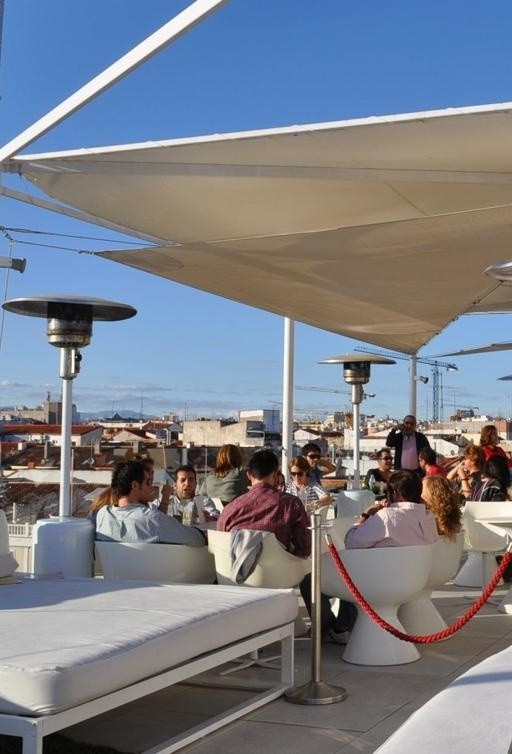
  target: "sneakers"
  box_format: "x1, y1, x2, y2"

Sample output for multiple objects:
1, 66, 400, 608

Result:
321, 629, 348, 645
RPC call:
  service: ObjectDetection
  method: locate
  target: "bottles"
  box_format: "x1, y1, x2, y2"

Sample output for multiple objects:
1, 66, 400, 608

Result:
346, 474, 379, 494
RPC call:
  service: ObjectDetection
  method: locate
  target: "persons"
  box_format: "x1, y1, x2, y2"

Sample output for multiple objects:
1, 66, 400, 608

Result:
273, 472, 287, 492
364, 413, 511, 584
421, 475, 463, 542
325, 468, 436, 645
85, 452, 175, 548
147, 465, 220, 524
89, 459, 211, 548
214, 448, 335, 639
198, 441, 251, 507
286, 456, 332, 523
301, 441, 336, 488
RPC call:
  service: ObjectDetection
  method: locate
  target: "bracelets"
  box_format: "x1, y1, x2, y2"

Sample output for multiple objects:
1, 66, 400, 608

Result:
160, 498, 169, 505
312, 500, 321, 507
360, 513, 369, 519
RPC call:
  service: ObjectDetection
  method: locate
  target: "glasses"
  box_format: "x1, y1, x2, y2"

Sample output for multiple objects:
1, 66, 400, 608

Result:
406, 422, 414, 425
290, 472, 304, 476
383, 456, 393, 460
307, 454, 321, 460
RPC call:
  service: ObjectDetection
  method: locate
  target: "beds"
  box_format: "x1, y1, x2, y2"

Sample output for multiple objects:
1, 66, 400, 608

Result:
0, 563, 299, 754
